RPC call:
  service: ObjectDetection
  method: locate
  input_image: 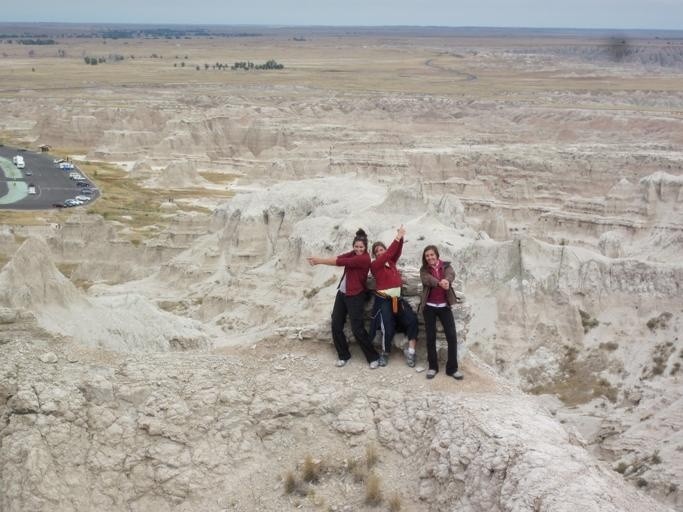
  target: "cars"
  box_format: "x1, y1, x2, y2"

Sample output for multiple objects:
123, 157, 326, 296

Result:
53, 158, 98, 208
13, 155, 25, 169
23, 170, 33, 176
28, 183, 36, 195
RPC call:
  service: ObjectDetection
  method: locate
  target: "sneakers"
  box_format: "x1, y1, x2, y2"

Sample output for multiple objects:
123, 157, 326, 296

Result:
338, 354, 351, 367
427, 369, 436, 378
370, 357, 380, 369
404, 349, 416, 367
446, 370, 464, 379
379, 352, 389, 366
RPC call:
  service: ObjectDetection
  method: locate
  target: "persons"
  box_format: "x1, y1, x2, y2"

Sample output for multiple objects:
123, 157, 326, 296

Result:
368, 220, 419, 366
306, 227, 379, 368
416, 242, 465, 380
